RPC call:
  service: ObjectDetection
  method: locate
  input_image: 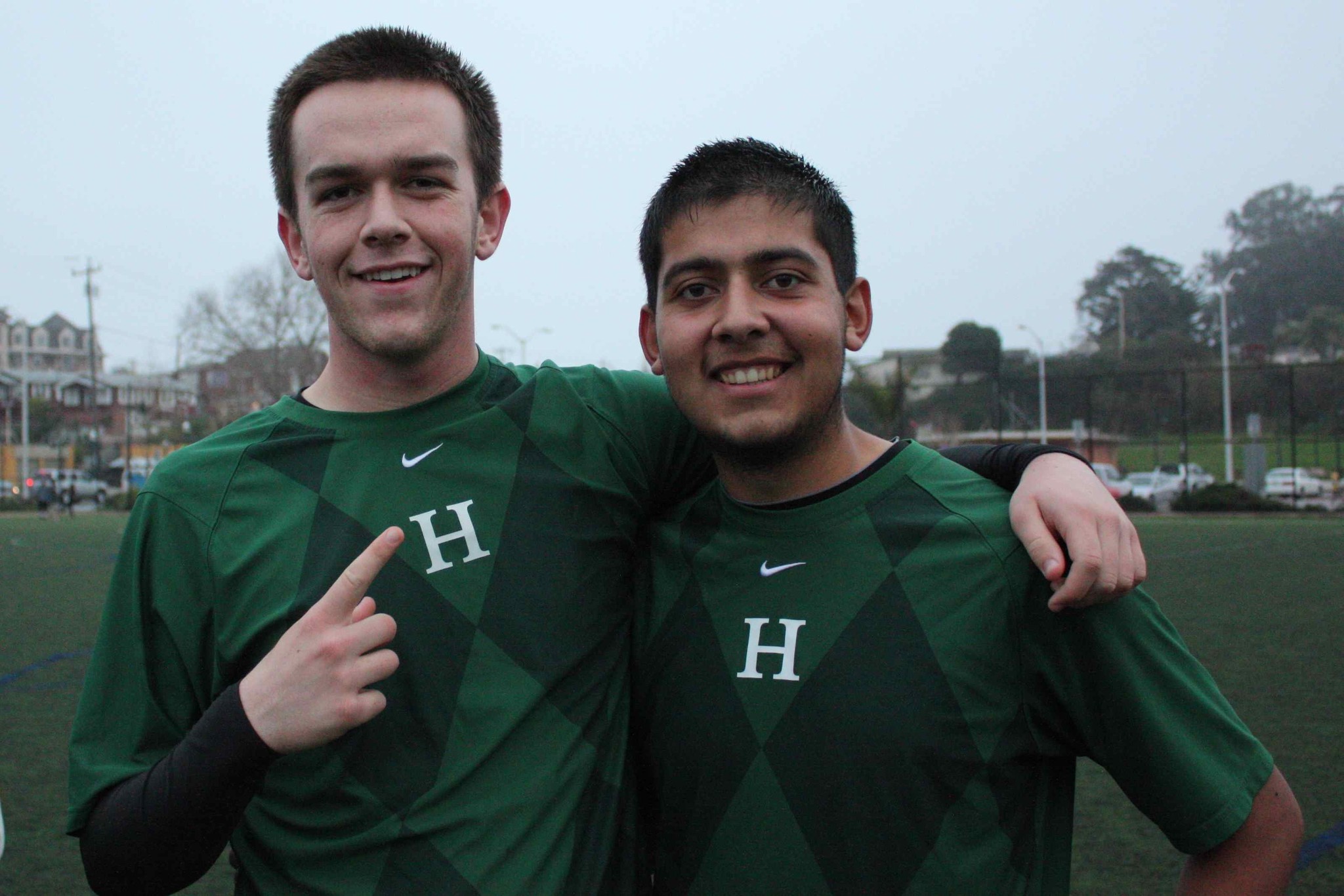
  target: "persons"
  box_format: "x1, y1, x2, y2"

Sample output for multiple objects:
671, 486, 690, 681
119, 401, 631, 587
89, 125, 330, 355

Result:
229, 137, 1305, 896
66, 21, 1150, 896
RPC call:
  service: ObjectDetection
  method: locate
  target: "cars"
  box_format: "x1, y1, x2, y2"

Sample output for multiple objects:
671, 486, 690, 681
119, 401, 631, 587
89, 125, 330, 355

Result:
1089, 459, 1343, 506
0, 455, 165, 507
